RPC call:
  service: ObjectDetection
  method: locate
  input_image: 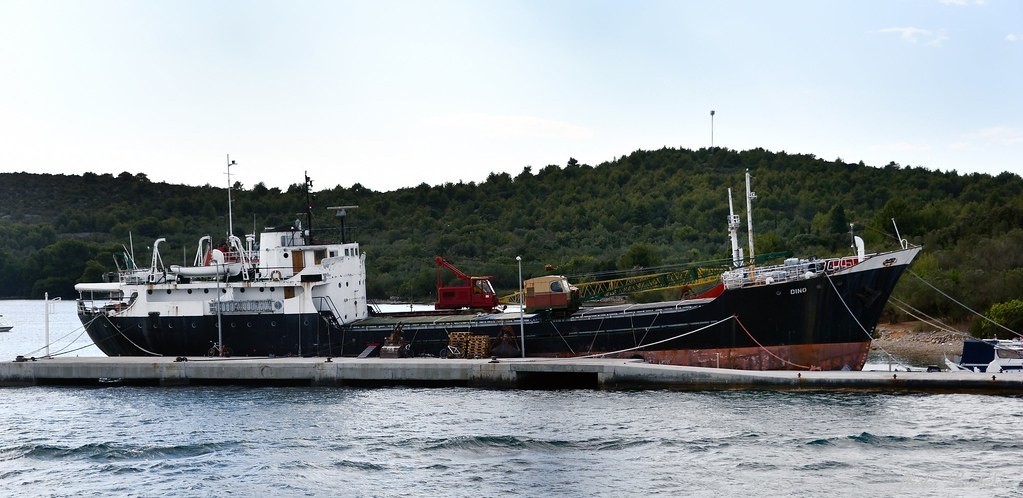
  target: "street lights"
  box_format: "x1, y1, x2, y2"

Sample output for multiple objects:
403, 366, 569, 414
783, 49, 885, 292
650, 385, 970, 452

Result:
516, 256, 524, 358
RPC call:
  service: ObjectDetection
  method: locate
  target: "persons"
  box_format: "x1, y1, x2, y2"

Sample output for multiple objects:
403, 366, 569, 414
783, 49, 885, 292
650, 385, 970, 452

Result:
79, 301, 101, 315
295, 215, 301, 230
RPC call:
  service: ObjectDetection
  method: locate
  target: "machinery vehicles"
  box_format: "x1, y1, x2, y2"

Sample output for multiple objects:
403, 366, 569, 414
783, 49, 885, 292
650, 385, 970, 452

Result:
434, 256, 502, 313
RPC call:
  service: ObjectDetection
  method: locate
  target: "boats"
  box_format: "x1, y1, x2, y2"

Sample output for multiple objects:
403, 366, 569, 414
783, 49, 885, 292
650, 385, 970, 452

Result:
76, 154, 924, 371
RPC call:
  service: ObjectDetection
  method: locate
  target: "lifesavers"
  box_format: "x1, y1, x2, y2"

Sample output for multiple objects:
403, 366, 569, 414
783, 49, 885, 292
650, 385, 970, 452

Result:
270, 270, 282, 281
